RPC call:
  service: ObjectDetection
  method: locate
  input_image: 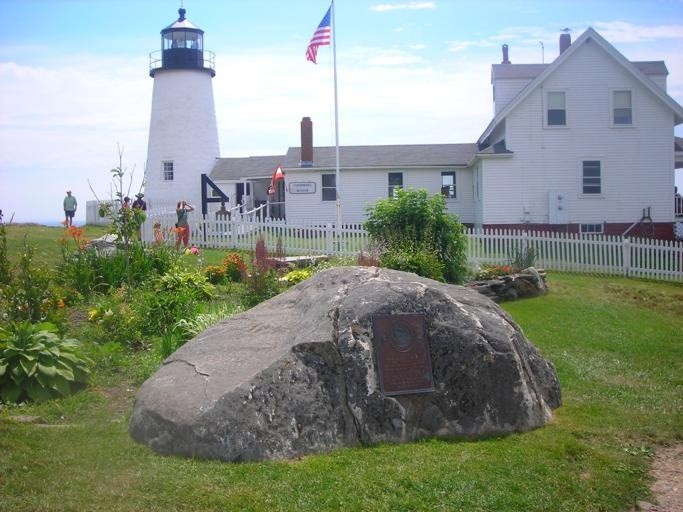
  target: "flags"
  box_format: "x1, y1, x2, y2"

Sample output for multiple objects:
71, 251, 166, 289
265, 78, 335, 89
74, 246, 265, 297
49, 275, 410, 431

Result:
270, 165, 283, 190
304, 4, 333, 64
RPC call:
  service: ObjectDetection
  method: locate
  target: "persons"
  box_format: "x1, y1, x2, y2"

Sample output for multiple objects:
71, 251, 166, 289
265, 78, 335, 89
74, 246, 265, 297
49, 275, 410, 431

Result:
123, 196, 130, 208
153, 222, 163, 244
63, 189, 77, 227
132, 193, 146, 240
176, 200, 194, 250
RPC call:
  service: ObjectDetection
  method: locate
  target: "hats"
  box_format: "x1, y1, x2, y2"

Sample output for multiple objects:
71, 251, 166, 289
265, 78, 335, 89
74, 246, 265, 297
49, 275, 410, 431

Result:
135, 193, 144, 198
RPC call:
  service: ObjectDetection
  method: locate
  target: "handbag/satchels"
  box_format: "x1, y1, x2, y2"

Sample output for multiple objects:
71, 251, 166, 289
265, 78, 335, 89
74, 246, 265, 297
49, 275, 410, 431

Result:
182, 245, 200, 255
